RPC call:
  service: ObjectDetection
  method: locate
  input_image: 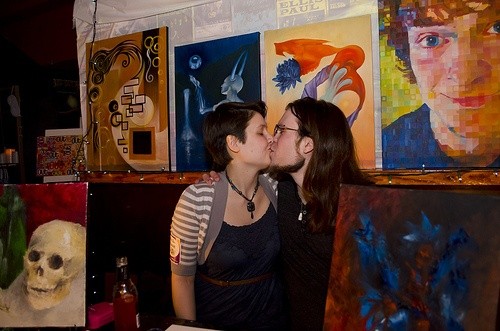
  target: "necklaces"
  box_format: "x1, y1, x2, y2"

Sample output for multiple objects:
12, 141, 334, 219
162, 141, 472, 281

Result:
225, 170, 260, 219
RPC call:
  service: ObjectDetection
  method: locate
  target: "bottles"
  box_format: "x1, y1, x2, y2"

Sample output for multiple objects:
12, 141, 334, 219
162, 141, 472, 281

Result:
112, 257, 140, 331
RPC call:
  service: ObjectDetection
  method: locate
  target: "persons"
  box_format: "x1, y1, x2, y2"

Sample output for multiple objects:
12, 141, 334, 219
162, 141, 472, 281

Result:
193, 96, 375, 331
168, 101, 278, 331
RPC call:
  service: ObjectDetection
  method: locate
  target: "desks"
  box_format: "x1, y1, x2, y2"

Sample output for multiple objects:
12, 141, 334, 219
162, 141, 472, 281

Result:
90, 310, 222, 331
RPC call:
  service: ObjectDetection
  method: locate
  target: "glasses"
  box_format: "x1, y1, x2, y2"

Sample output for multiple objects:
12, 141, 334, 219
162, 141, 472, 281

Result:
274, 124, 298, 136
